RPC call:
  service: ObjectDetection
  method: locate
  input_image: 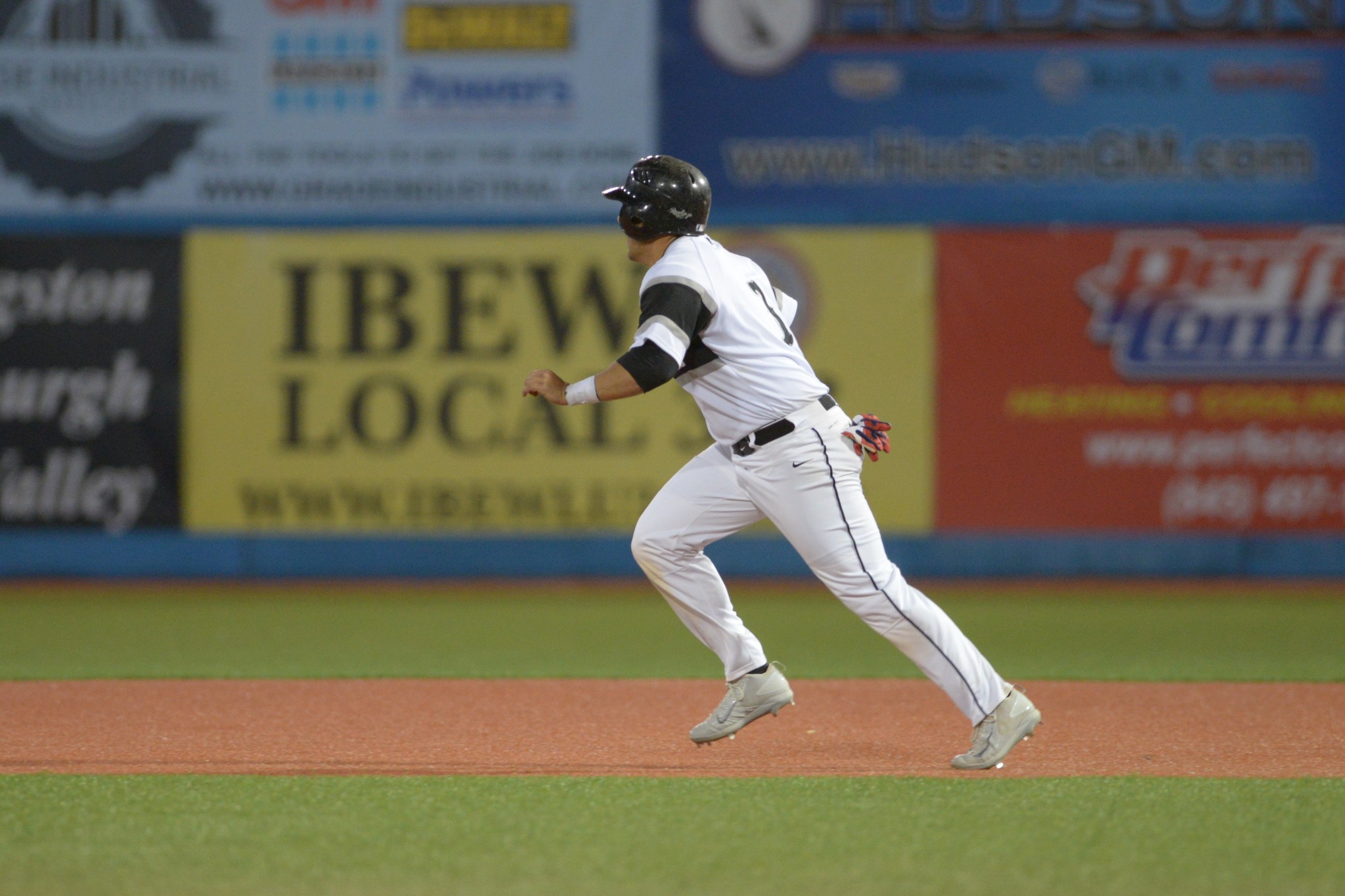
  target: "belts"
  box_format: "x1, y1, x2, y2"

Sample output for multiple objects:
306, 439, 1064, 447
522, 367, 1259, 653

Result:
732, 394, 835, 455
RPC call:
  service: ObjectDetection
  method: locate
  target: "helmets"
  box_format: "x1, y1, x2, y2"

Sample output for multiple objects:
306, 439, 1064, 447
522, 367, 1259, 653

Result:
602, 152, 712, 241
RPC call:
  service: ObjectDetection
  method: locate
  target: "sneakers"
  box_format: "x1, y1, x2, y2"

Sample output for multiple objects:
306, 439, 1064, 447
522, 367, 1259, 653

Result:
688, 662, 794, 747
951, 687, 1043, 769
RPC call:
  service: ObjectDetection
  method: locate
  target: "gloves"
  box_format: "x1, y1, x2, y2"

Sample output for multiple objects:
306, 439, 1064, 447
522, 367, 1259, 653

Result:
842, 413, 892, 462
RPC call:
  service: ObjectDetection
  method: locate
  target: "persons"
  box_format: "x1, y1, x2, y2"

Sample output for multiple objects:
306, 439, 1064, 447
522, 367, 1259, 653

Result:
521, 155, 1044, 770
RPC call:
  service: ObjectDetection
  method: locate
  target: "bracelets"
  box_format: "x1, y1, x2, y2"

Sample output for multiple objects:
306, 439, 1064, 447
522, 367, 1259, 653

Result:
565, 375, 601, 407
